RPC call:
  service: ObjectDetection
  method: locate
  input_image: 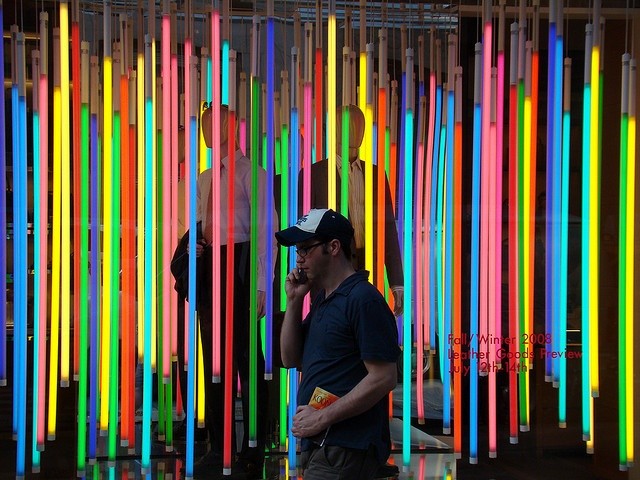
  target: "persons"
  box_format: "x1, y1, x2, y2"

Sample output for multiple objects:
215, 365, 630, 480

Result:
297, 105, 404, 320
185, 103, 282, 471
274, 209, 399, 480
179, 124, 184, 238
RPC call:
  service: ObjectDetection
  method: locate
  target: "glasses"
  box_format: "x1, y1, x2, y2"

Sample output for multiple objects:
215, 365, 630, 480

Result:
295, 240, 329, 258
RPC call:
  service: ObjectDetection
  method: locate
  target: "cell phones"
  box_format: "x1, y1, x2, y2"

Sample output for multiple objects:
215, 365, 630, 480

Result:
295, 269, 307, 284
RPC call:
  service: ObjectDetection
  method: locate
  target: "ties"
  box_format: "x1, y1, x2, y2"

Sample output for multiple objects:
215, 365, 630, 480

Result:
203, 162, 224, 247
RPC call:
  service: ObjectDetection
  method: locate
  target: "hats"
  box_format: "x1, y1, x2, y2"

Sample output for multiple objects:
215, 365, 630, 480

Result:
274, 208, 354, 247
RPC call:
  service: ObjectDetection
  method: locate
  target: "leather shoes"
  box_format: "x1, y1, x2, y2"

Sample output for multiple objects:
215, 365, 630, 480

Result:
179, 449, 224, 473
228, 448, 264, 476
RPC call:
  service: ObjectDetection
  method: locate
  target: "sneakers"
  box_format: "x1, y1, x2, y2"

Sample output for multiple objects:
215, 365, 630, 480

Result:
154, 426, 209, 443
